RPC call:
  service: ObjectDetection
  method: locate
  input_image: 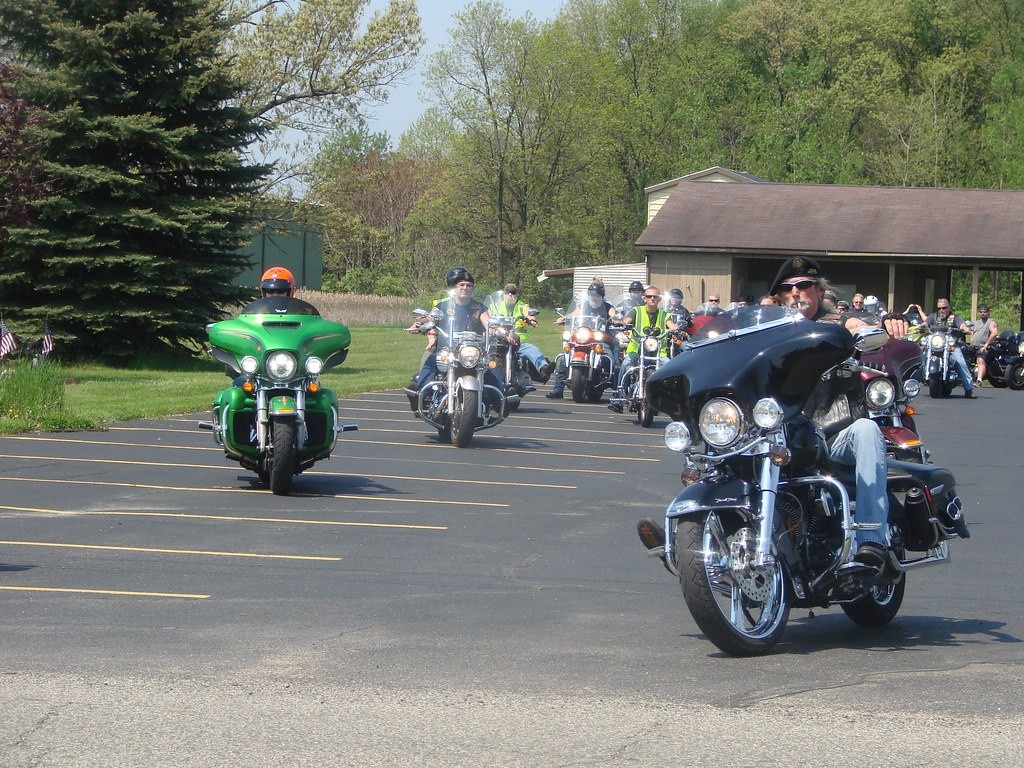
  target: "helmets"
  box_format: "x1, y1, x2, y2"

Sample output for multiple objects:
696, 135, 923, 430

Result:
447, 268, 466, 287
669, 289, 683, 299
262, 266, 295, 298
629, 281, 643, 293
588, 283, 605, 296
863, 296, 879, 314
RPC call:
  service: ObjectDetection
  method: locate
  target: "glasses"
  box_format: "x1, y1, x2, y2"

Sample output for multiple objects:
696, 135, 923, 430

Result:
855, 302, 863, 303
459, 283, 474, 287
779, 280, 819, 292
263, 289, 287, 294
937, 306, 948, 309
709, 300, 718, 302
646, 295, 659, 298
505, 292, 516, 295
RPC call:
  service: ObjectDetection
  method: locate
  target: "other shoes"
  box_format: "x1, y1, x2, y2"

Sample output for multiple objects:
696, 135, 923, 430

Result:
973, 380, 982, 388
406, 382, 419, 411
540, 362, 556, 383
637, 517, 677, 575
608, 403, 623, 413
499, 387, 517, 418
965, 391, 978, 398
857, 542, 890, 567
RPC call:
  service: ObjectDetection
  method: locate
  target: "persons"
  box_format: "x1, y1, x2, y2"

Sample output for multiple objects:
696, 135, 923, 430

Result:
545, 277, 786, 414
405, 268, 517, 418
637, 255, 910, 576
488, 283, 556, 382
232, 266, 320, 387
812, 290, 998, 399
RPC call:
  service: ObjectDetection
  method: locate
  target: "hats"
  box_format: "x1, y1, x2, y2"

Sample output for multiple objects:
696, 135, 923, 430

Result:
770, 256, 825, 297
837, 300, 849, 310
979, 305, 989, 312
452, 272, 474, 287
824, 290, 836, 303
504, 284, 518, 294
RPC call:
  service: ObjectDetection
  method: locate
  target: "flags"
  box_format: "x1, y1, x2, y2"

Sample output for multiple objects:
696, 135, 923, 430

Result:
42, 319, 55, 353
0, 319, 17, 356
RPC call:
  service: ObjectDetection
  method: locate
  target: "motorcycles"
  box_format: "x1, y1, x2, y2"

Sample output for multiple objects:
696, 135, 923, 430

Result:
643, 302, 971, 657
402, 286, 518, 448
608, 284, 1024, 426
552, 290, 623, 404
485, 309, 540, 411
196, 296, 360, 494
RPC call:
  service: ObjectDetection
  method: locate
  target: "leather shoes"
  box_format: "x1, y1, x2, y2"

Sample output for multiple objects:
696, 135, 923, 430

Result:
546, 390, 564, 398
